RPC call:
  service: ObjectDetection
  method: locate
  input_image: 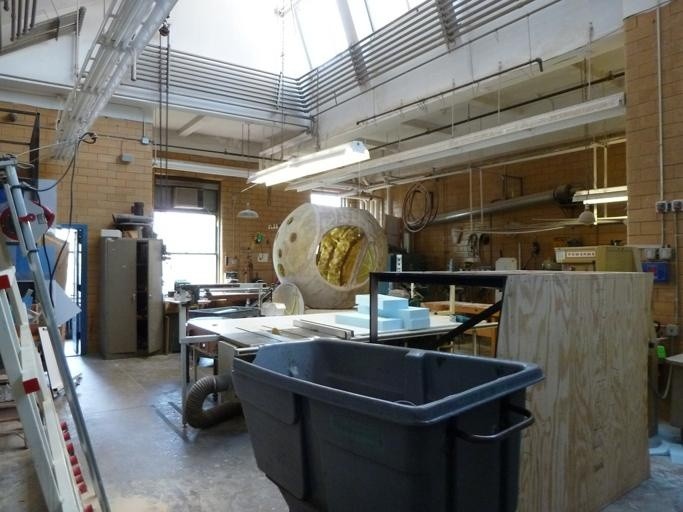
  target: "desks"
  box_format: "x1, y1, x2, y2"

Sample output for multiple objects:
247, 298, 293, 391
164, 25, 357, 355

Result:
180, 308, 499, 425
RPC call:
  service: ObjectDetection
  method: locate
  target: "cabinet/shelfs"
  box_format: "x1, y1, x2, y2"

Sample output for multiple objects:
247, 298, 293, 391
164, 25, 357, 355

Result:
100, 237, 163, 359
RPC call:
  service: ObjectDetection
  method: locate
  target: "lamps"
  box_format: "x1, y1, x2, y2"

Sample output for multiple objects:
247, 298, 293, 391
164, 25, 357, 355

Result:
235, 1, 371, 187
235, 122, 259, 219
572, 56, 628, 205
